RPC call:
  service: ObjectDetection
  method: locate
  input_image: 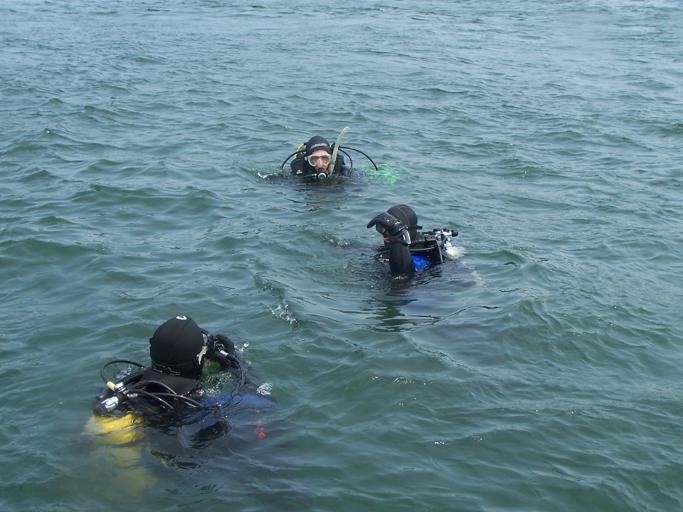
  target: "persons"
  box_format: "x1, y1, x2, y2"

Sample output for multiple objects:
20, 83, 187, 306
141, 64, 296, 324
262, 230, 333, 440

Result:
99, 313, 289, 478
264, 133, 371, 191
367, 202, 453, 286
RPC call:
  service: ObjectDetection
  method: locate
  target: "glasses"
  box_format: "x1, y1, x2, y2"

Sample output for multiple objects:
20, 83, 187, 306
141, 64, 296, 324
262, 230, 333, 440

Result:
306, 153, 331, 167
